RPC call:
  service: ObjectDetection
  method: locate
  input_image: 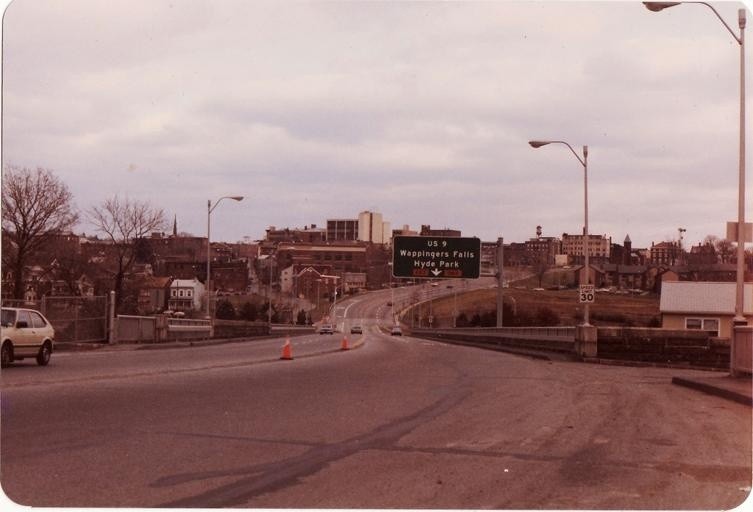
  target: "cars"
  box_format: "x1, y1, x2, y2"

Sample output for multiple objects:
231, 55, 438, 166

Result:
359, 289, 368, 295
386, 300, 395, 306
161, 309, 174, 317
0, 306, 55, 369
430, 281, 440, 287
172, 311, 184, 319
390, 326, 402, 336
350, 325, 362, 334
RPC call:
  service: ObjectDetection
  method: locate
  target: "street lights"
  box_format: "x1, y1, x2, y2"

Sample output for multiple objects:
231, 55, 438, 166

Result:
381, 283, 405, 317
318, 274, 349, 331
267, 246, 295, 335
639, 2, 749, 379
527, 138, 598, 356
204, 195, 244, 322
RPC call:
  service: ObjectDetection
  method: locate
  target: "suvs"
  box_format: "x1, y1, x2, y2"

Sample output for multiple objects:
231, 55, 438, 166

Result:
318, 322, 334, 335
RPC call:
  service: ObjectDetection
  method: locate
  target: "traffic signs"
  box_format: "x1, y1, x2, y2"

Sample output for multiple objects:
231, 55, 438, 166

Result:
390, 234, 481, 279
578, 283, 595, 304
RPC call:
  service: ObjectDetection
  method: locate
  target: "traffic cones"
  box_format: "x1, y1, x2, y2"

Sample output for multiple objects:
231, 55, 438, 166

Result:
340, 334, 350, 351
279, 333, 293, 360
